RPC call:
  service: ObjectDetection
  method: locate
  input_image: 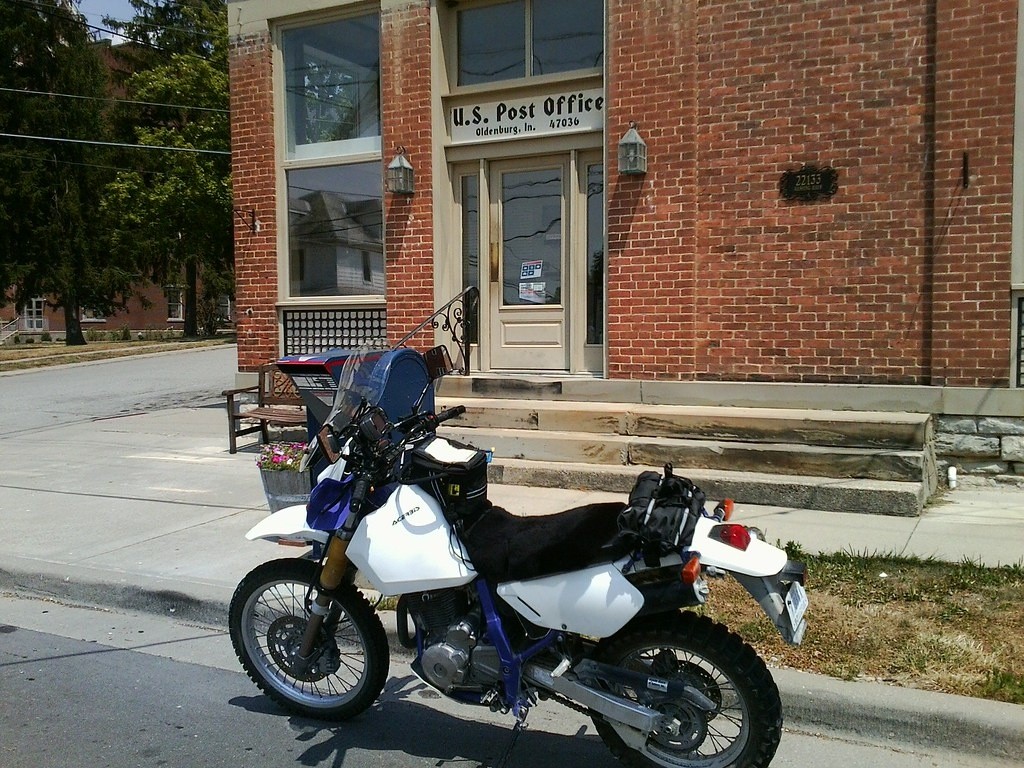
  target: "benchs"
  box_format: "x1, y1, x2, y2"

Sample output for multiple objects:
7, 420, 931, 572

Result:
220, 363, 308, 455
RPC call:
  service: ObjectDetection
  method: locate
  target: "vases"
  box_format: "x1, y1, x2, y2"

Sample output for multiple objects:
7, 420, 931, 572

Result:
260, 470, 311, 515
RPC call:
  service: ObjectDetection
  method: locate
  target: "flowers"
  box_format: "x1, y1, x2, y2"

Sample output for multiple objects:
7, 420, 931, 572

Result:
256, 440, 310, 471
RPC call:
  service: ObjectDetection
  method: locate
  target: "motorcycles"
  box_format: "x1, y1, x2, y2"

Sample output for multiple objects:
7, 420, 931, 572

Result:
224, 333, 811, 768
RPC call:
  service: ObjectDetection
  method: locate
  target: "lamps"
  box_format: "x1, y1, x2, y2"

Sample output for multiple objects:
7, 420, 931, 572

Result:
387, 147, 414, 194
617, 121, 648, 174
